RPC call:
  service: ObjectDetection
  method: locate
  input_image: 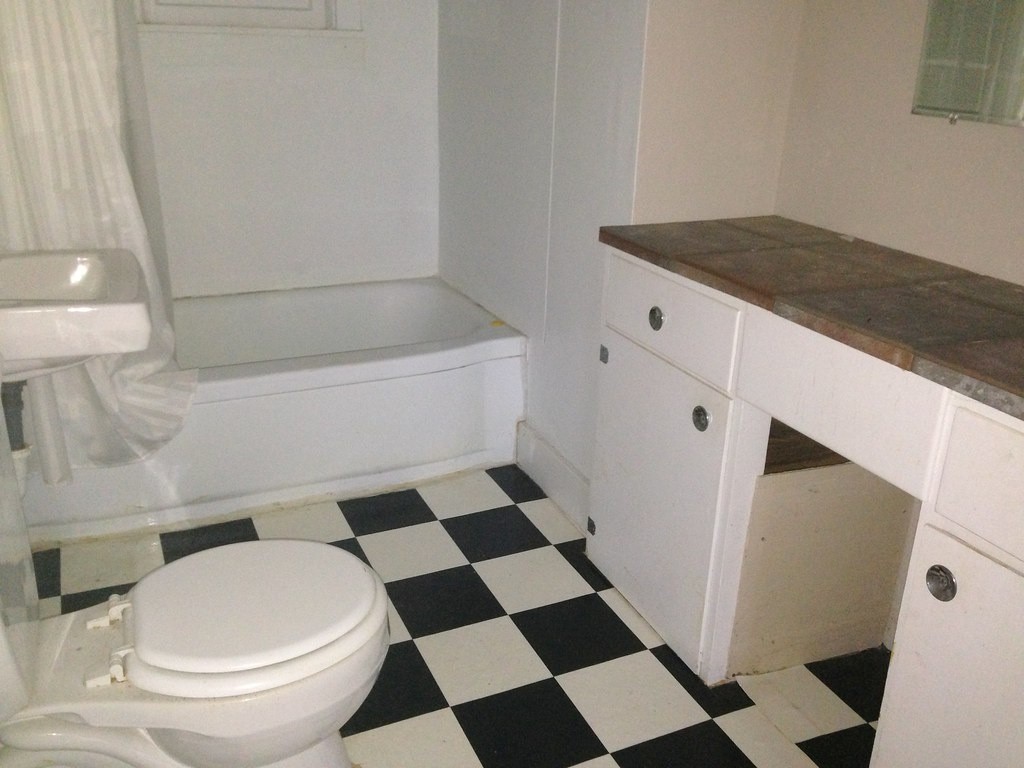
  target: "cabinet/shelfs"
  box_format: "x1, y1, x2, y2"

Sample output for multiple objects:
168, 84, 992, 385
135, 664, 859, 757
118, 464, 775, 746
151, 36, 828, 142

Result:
588, 215, 1023, 767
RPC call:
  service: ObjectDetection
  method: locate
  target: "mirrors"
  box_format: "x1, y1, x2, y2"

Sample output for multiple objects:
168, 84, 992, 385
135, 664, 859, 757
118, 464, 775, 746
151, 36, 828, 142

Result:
909, 0, 1024, 128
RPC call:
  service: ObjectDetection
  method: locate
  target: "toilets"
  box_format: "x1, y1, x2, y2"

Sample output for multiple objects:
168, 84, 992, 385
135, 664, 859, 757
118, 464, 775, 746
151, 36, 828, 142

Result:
0, 537, 390, 762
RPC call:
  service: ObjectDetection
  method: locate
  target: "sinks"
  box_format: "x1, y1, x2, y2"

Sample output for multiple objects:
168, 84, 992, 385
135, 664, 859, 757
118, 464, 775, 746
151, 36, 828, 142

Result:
1, 247, 153, 386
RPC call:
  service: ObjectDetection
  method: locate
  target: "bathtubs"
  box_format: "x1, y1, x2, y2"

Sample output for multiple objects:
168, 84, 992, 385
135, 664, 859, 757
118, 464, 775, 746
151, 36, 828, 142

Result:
13, 275, 529, 547
